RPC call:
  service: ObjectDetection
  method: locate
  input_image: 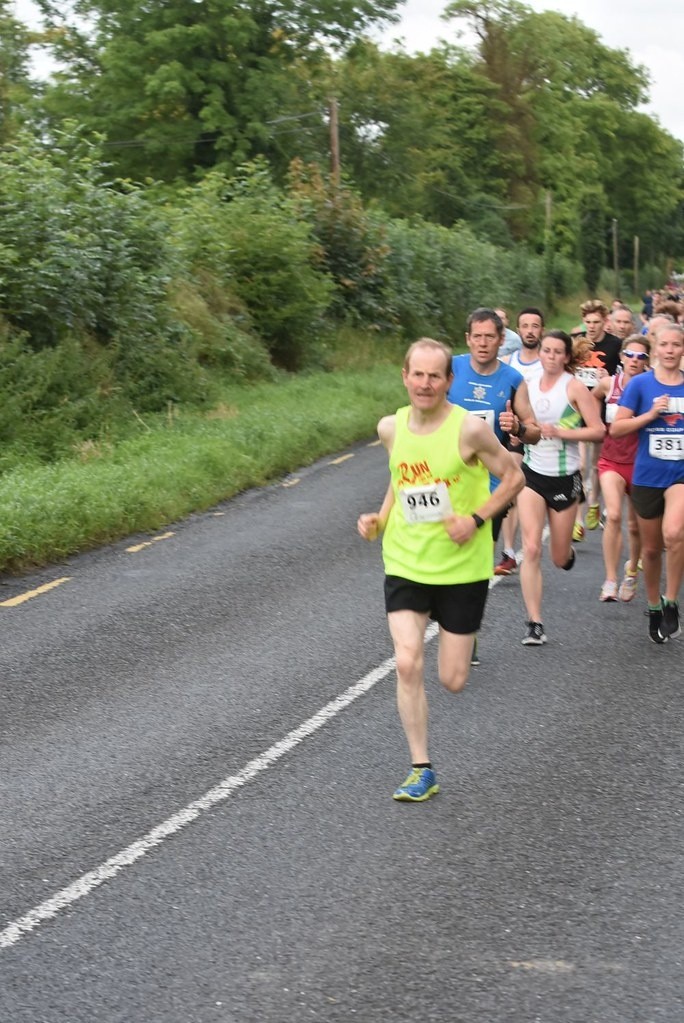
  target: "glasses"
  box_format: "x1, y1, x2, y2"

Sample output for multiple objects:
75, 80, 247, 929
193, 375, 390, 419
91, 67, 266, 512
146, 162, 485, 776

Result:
622, 349, 649, 360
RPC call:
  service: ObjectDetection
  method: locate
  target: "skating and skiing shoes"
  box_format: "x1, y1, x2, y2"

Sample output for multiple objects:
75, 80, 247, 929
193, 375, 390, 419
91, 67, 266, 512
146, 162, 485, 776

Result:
521, 621, 548, 645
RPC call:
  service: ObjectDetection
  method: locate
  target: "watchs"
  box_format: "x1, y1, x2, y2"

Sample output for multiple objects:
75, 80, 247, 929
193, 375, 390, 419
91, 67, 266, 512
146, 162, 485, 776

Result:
471, 513, 485, 528
512, 422, 526, 438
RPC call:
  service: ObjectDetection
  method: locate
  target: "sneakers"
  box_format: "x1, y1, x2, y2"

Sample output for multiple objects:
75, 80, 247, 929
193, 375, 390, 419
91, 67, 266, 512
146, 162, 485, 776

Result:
618, 560, 640, 602
471, 632, 481, 666
646, 594, 682, 644
561, 545, 578, 571
585, 503, 607, 531
495, 551, 519, 575
599, 580, 618, 602
572, 520, 585, 541
392, 767, 440, 802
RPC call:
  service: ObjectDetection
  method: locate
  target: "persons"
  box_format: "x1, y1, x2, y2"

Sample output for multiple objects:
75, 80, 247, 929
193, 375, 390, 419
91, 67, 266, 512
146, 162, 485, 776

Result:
493, 307, 545, 575
516, 329, 606, 645
357, 336, 527, 802
446, 308, 541, 664
494, 283, 684, 371
567, 299, 622, 542
590, 333, 650, 603
609, 324, 684, 645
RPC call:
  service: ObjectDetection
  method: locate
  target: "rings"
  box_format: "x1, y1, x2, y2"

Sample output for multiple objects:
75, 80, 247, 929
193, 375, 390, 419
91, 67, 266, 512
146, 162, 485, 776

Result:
504, 422, 506, 426
